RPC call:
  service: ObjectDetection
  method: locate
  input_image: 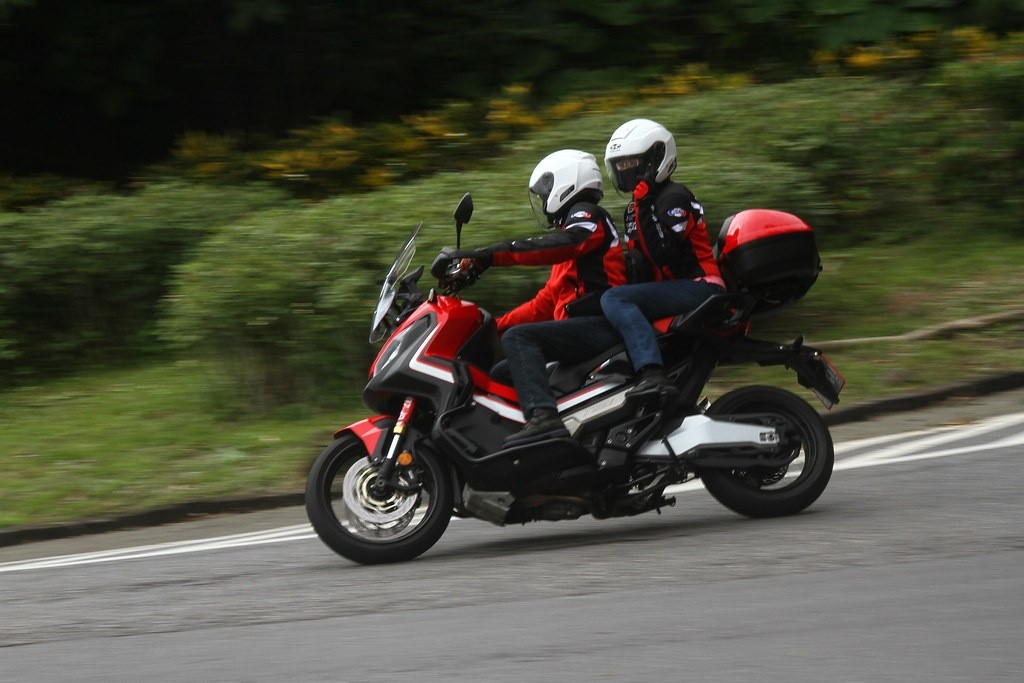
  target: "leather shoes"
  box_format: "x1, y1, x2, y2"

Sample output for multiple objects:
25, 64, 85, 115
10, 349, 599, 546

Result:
501, 407, 570, 450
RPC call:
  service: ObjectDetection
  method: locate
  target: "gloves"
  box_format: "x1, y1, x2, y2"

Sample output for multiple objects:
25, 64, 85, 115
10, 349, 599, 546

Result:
442, 247, 493, 286
634, 181, 650, 199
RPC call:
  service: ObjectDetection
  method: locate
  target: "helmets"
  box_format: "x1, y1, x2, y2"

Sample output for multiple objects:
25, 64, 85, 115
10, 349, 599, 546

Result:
529, 149, 604, 214
604, 119, 677, 192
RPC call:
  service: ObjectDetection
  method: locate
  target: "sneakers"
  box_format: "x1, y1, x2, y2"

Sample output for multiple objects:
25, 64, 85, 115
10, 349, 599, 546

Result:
625, 367, 680, 399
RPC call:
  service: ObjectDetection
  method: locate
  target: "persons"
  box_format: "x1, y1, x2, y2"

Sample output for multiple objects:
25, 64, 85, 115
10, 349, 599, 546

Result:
447, 149, 628, 449
600, 119, 727, 400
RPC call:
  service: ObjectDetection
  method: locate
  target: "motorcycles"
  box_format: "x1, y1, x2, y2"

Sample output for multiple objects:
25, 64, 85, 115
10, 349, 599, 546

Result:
307, 191, 849, 564
